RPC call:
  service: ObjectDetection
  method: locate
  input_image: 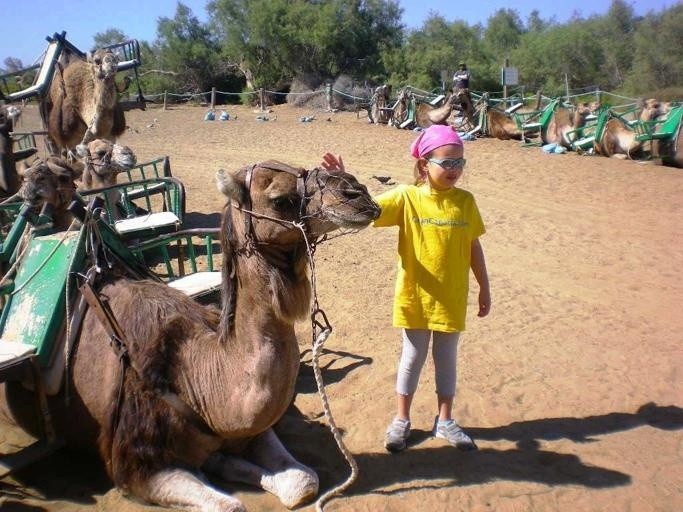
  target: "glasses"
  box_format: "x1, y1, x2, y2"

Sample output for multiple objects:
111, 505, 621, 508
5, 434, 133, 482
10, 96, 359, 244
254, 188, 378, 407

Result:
429, 158, 467, 169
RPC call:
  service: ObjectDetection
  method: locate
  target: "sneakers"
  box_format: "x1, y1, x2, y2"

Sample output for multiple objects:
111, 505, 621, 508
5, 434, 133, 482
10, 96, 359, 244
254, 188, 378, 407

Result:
432, 415, 475, 451
384, 414, 411, 452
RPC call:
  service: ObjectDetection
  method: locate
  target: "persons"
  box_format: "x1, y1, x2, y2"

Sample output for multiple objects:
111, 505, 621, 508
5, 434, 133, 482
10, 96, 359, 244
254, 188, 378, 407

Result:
451, 61, 471, 92
320, 123, 491, 451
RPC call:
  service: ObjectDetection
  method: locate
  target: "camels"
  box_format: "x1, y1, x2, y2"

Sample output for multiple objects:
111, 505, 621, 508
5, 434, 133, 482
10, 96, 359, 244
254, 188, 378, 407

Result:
643, 106, 683, 169
593, 98, 670, 161
0, 159, 381, 512
369, 82, 539, 145
370, 83, 682, 170
540, 96, 601, 150
0, 48, 137, 272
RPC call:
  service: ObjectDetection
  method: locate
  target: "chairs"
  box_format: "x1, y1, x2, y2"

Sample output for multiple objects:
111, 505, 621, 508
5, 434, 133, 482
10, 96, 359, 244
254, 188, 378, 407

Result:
1, 155, 225, 475
0, 32, 146, 136
513, 95, 679, 154
